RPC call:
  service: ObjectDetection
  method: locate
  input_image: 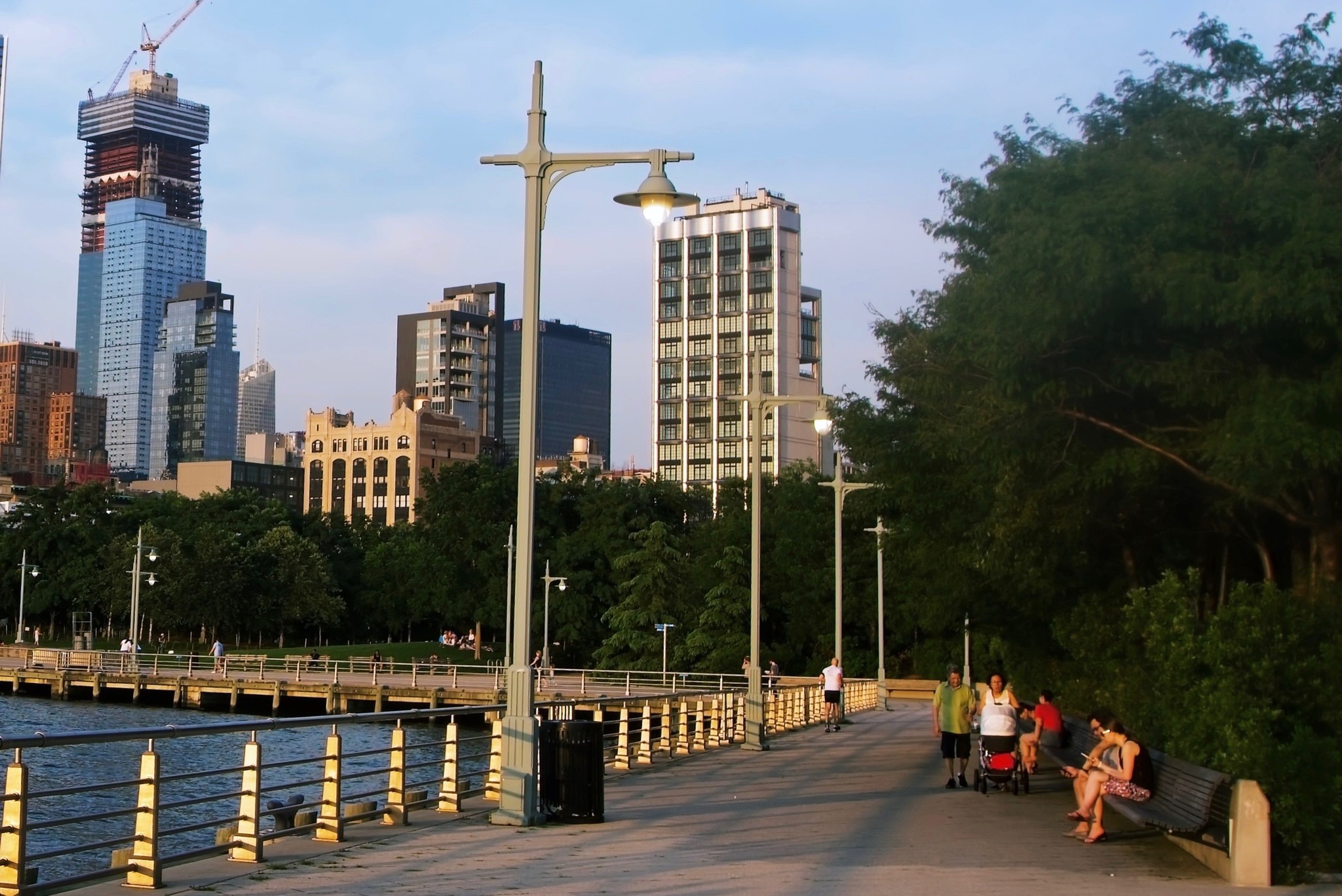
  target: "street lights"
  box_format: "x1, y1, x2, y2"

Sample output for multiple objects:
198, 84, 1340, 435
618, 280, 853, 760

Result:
14, 549, 42, 643
473, 61, 704, 827
724, 352, 839, 754
540, 558, 570, 677
131, 525, 161, 673
125, 554, 159, 640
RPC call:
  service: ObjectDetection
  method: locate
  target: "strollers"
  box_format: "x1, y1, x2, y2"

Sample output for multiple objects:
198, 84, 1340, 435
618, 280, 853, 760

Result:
973, 703, 1030, 797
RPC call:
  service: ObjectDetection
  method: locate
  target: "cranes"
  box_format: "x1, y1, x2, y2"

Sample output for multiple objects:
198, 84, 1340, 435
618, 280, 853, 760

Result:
87, 50, 138, 106
139, 0, 205, 72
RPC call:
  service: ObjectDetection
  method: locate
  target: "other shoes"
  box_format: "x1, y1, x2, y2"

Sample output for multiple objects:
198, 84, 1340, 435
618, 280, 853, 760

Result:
945, 778, 956, 789
1061, 827, 1090, 840
957, 772, 968, 788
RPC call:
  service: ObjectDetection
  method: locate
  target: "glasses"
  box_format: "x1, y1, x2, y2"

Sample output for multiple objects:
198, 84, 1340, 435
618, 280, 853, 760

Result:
1092, 724, 1101, 732
1100, 729, 1110, 737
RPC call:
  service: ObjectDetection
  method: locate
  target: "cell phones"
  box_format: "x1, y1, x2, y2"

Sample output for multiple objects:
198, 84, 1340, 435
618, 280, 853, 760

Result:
1058, 766, 1072, 776
1081, 753, 1094, 762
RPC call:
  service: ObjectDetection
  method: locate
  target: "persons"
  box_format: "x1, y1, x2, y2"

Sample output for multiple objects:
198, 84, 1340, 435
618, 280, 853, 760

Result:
529, 650, 548, 688
932, 664, 977, 789
369, 650, 384, 673
819, 657, 843, 733
442, 629, 476, 650
766, 658, 779, 697
208, 638, 224, 674
158, 633, 165, 654
119, 637, 142, 664
742, 656, 751, 687
34, 627, 44, 645
548, 664, 557, 685
306, 649, 320, 673
1060, 708, 1154, 843
978, 670, 1019, 715
1015, 689, 1063, 774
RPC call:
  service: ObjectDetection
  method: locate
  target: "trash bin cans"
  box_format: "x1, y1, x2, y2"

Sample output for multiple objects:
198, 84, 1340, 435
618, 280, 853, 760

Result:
539, 718, 605, 824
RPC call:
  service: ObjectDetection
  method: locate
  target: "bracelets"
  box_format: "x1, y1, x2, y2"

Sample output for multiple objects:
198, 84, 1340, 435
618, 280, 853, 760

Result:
1097, 761, 1102, 768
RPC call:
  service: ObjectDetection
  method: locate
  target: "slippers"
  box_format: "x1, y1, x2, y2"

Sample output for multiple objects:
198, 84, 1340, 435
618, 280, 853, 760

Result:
1067, 811, 1091, 822
1083, 831, 1109, 844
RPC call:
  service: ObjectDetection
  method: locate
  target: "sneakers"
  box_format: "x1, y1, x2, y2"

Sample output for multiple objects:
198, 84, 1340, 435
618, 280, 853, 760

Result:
833, 725, 841, 732
825, 723, 830, 731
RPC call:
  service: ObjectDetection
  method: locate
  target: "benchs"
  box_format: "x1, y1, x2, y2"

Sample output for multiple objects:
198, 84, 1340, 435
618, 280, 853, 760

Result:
886, 678, 941, 695
1014, 700, 1233, 834
104, 649, 128, 665
411, 656, 453, 673
283, 655, 330, 672
225, 653, 267, 670
349, 655, 395, 675
